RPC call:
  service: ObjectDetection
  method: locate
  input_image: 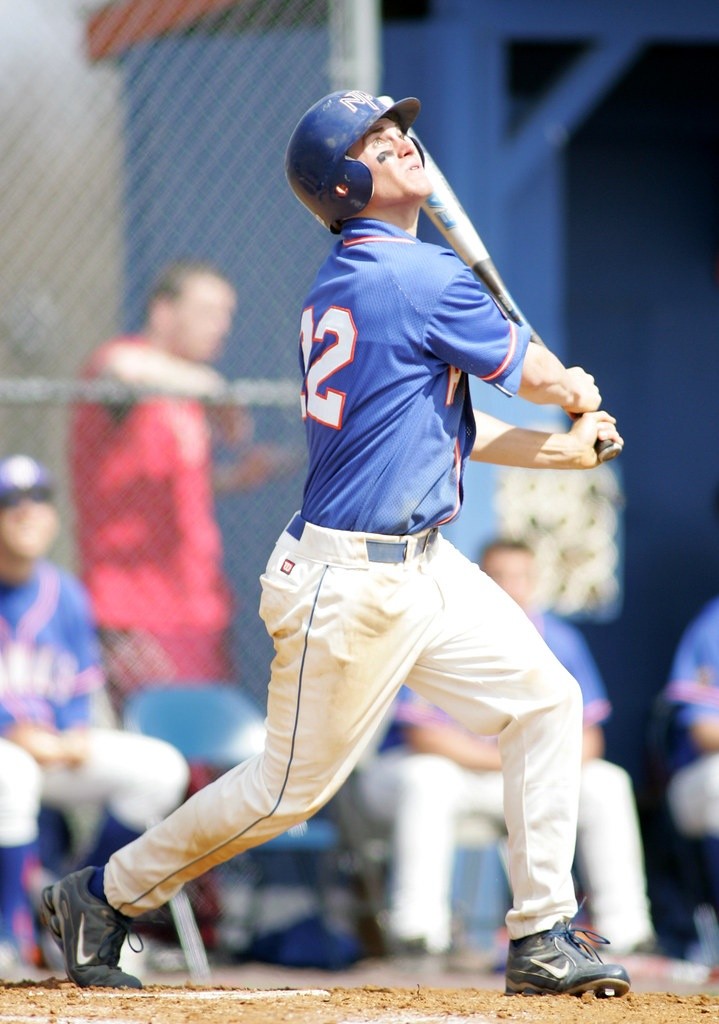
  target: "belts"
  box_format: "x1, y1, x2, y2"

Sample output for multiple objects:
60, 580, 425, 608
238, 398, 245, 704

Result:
284, 513, 438, 562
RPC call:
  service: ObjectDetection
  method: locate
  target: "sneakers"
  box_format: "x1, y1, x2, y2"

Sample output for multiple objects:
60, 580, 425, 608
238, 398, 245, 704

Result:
40, 866, 145, 990
504, 896, 630, 996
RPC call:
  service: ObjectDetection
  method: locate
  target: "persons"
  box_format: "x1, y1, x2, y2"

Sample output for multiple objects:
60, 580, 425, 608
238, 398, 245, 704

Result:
0, 454, 188, 976
38, 90, 631, 1003
356, 540, 652, 982
67, 269, 275, 956
665, 603, 719, 832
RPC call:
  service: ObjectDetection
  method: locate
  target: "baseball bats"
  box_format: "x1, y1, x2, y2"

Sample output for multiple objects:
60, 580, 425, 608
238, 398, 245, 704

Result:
406, 128, 624, 465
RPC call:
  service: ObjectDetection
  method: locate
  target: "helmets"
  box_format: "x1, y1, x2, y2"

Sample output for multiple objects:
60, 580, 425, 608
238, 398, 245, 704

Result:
286, 90, 422, 234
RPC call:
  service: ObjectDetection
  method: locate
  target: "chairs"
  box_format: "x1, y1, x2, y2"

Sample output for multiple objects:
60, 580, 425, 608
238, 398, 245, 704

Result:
125, 682, 409, 986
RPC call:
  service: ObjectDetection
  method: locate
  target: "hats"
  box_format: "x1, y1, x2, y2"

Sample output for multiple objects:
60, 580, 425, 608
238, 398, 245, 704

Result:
0, 454, 54, 500
